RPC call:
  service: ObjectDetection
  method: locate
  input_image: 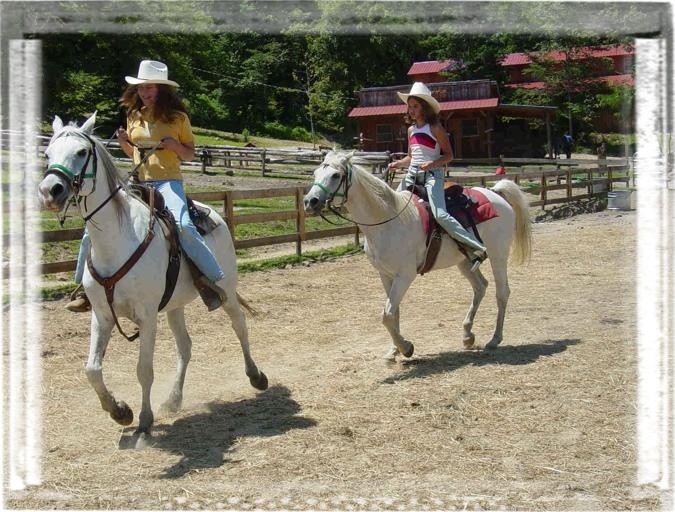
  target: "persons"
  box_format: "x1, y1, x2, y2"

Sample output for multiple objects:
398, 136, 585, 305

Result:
389, 82, 487, 272
65, 60, 226, 311
552, 134, 562, 159
562, 132, 573, 158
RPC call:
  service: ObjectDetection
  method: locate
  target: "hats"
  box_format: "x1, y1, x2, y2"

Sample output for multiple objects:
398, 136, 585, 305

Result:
124, 59, 180, 87
397, 81, 441, 115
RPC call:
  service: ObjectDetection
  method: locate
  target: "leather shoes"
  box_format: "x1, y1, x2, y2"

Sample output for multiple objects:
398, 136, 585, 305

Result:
65, 298, 89, 312
208, 294, 221, 311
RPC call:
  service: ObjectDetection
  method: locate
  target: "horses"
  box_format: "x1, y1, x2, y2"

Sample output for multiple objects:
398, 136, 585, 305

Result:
37, 109, 269, 436
302, 140, 534, 365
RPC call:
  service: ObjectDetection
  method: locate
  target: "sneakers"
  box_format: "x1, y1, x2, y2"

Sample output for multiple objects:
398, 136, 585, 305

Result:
472, 252, 487, 264
